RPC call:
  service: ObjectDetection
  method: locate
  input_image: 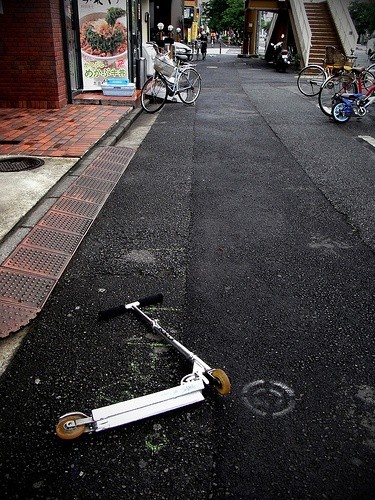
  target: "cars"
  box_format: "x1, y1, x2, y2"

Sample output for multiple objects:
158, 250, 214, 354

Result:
161, 36, 198, 61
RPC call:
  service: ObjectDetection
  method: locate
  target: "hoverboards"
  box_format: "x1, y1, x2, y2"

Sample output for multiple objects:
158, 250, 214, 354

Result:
56, 292, 231, 440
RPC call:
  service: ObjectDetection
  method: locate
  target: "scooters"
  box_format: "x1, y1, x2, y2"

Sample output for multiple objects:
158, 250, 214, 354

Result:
270, 33, 290, 73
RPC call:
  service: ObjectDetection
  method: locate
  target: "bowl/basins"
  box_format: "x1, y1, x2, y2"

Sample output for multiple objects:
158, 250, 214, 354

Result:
80, 12, 127, 60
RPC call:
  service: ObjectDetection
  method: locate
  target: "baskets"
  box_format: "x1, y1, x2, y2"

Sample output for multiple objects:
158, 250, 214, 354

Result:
154, 56, 175, 78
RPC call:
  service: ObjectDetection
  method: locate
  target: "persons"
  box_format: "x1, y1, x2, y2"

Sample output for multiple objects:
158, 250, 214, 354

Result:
196, 31, 208, 61
210, 30, 216, 48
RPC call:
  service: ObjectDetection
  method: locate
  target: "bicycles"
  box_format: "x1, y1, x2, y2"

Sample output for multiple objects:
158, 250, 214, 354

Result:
296, 45, 375, 124
139, 41, 202, 114
